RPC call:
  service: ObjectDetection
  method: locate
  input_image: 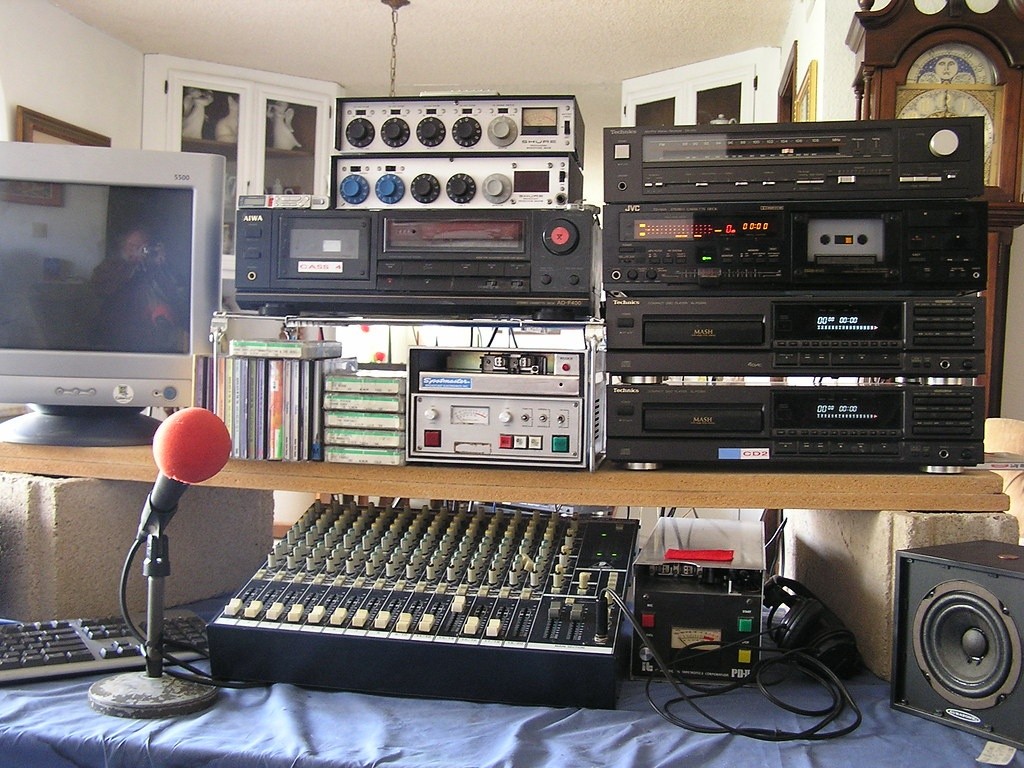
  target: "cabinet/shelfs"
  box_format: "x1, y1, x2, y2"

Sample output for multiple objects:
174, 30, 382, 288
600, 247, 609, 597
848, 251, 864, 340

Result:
0, 442, 1024, 768
165, 74, 329, 272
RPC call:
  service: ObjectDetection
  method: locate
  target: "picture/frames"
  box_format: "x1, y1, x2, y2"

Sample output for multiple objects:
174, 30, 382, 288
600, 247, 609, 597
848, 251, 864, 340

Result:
794, 58, 817, 122
17, 105, 112, 147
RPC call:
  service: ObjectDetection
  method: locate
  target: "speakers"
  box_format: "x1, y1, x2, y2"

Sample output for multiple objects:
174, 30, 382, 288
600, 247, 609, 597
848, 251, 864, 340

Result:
891, 539, 1024, 750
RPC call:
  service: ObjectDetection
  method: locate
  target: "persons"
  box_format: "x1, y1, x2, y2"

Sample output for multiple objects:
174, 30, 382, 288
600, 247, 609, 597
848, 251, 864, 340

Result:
69, 220, 188, 352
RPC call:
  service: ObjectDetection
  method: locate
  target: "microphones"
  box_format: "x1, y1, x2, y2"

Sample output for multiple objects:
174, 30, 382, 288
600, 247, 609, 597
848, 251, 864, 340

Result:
135, 408, 231, 542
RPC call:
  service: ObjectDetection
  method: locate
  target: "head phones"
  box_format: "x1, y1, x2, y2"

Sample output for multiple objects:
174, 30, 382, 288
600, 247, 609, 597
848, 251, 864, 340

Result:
762, 575, 861, 677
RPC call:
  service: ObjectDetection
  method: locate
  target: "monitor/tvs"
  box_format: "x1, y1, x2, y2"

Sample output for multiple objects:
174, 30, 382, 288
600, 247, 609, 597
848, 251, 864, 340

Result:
0, 142, 226, 448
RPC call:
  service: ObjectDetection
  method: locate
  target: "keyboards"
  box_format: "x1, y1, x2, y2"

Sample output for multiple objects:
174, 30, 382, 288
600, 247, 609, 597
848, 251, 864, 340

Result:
0, 609, 211, 682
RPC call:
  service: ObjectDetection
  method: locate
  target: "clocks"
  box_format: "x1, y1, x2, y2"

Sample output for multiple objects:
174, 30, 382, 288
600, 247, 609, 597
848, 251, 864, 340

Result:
846, 0, 1024, 421
